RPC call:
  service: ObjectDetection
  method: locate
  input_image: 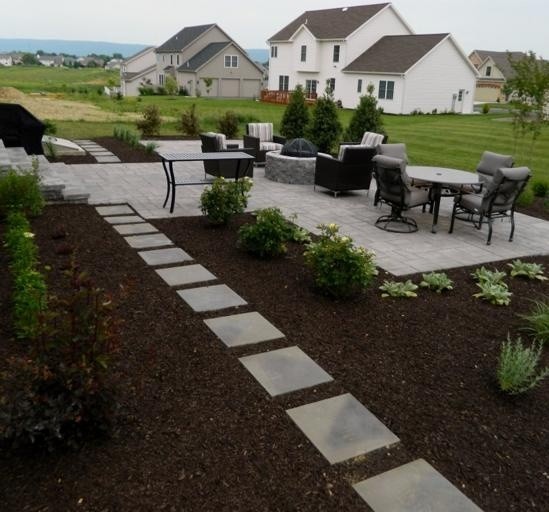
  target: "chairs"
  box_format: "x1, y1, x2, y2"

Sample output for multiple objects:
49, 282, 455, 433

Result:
451, 150, 516, 222
337, 130, 389, 177
372, 155, 432, 233
314, 144, 375, 197
200, 130, 255, 179
375, 142, 428, 213
243, 122, 288, 167
449, 166, 533, 245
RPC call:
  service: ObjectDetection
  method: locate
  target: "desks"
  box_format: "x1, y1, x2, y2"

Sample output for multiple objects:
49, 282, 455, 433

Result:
155, 152, 255, 213
405, 164, 488, 234
